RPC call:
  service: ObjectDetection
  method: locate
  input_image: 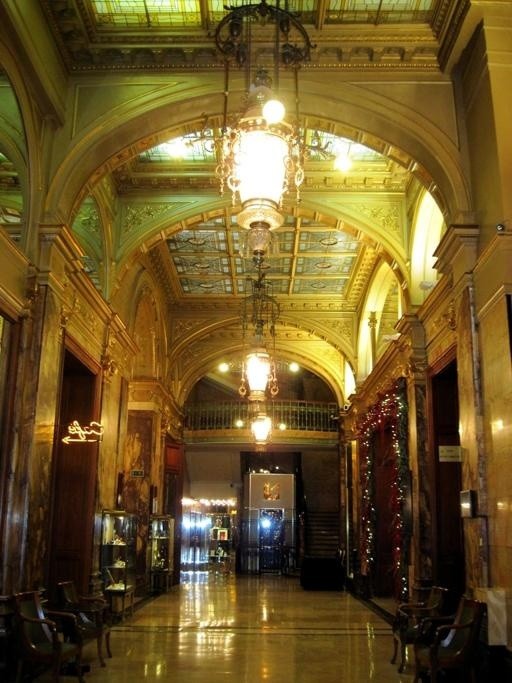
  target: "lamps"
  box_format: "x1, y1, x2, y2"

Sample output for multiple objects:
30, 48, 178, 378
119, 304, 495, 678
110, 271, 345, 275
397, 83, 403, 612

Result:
218, 269, 300, 403
233, 400, 288, 459
167, 0, 355, 264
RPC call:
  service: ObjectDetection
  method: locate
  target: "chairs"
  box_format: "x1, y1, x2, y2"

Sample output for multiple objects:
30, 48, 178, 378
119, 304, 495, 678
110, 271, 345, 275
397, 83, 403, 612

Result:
390, 585, 487, 683
11, 580, 114, 683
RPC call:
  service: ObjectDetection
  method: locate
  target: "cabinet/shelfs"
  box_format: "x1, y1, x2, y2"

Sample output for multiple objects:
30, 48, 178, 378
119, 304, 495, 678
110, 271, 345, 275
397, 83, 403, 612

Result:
149, 515, 175, 593
99, 508, 139, 621
208, 515, 232, 559
